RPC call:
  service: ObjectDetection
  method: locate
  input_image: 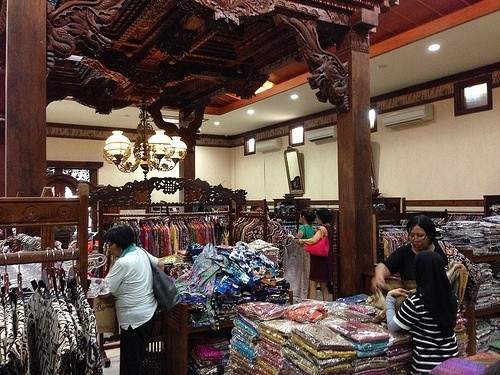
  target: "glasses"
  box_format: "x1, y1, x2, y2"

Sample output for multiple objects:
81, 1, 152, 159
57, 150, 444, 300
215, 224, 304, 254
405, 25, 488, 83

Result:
408, 233, 427, 241
106, 243, 114, 248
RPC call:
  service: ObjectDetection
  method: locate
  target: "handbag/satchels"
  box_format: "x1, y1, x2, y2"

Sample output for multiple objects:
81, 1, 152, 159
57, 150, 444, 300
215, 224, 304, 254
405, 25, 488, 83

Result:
304, 229, 329, 256
144, 251, 182, 311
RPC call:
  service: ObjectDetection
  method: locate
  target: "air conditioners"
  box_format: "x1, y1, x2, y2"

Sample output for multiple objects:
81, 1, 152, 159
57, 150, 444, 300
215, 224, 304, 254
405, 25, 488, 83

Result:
256, 137, 284, 153
382, 103, 436, 129
308, 126, 337, 142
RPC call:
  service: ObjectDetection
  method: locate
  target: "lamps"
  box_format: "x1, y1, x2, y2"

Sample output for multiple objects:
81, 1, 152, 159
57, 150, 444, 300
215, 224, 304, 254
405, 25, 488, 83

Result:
101, 99, 188, 175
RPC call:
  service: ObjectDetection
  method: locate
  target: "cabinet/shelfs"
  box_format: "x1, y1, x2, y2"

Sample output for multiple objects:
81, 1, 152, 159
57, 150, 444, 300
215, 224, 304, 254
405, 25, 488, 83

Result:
155, 282, 294, 375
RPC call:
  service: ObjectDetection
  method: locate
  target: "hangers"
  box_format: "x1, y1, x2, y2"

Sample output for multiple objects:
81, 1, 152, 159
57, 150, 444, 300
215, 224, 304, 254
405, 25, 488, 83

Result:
0, 232, 112, 298
120, 211, 264, 227
380, 211, 484, 236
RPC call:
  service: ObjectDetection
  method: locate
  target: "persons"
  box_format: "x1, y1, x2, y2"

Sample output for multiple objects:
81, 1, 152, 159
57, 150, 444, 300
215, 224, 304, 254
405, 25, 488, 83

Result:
89, 223, 166, 375
250, 206, 334, 303
375, 214, 468, 375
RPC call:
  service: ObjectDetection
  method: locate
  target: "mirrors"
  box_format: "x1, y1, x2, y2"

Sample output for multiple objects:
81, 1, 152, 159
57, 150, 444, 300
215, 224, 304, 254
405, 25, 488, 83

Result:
284, 147, 302, 197
